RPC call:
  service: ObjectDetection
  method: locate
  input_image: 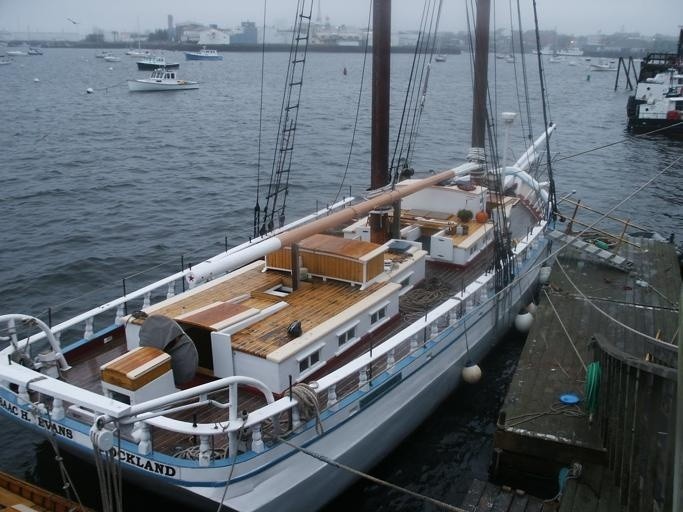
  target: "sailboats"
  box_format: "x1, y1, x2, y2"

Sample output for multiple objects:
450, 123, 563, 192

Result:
126, 37, 150, 56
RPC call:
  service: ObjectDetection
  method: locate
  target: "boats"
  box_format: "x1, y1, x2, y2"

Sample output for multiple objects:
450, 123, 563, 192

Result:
628, 27, 683, 132
589, 63, 617, 71
27, 47, 42, 55
497, 54, 515, 62
184, 49, 223, 60
126, 69, 199, 91
435, 54, 446, 61
7, 50, 22, 56
97, 51, 120, 62
0, 1, 558, 512
136, 55, 179, 70
533, 43, 583, 57
